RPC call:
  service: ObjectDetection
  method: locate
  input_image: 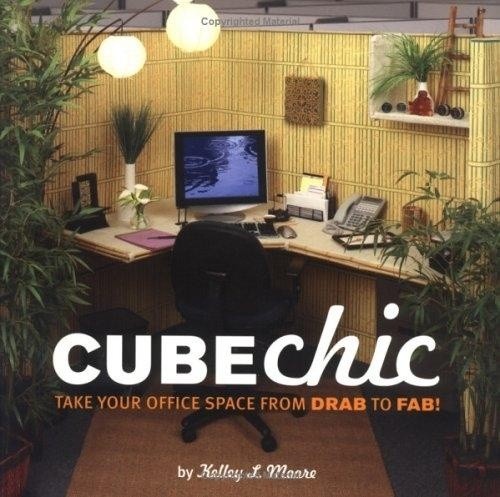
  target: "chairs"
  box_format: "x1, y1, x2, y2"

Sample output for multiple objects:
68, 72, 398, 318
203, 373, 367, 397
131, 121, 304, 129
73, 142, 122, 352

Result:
169, 220, 309, 452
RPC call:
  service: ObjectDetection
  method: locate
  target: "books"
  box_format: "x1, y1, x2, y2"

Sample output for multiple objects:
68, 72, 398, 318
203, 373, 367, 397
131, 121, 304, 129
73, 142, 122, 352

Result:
114, 228, 176, 251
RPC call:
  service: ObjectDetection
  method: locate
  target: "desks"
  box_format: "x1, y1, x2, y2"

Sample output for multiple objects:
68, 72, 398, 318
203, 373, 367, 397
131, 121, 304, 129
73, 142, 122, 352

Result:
57, 196, 464, 298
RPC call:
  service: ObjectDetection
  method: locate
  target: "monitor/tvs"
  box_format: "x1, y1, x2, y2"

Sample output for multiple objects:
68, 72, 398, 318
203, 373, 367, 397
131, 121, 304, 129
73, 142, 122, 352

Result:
173, 130, 267, 222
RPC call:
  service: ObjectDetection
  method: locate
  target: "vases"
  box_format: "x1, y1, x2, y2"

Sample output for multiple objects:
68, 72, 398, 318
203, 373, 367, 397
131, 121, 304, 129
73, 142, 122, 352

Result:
128, 208, 151, 228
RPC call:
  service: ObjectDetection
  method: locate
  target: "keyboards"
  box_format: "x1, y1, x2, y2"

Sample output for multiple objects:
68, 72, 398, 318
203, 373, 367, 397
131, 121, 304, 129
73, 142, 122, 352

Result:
232, 221, 280, 239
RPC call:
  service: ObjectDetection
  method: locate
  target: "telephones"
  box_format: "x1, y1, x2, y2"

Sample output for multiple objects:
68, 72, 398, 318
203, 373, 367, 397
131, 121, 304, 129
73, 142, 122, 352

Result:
333, 192, 388, 234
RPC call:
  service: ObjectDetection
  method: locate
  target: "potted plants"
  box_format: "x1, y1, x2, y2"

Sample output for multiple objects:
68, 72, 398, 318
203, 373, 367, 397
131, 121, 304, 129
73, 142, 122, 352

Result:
366, 29, 464, 115
107, 97, 165, 220
1, 0, 111, 495
343, 168, 500, 496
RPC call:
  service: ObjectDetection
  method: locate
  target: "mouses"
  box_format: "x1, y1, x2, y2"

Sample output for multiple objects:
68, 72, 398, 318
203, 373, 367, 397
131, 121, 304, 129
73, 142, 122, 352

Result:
278, 225, 297, 238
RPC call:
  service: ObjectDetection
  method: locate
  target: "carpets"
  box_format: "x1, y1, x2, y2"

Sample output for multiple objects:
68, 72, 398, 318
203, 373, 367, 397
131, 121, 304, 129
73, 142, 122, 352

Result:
67, 354, 397, 496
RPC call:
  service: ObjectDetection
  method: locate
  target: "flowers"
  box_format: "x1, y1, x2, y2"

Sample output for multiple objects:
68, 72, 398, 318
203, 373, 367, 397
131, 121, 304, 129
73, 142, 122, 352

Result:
115, 182, 159, 225
